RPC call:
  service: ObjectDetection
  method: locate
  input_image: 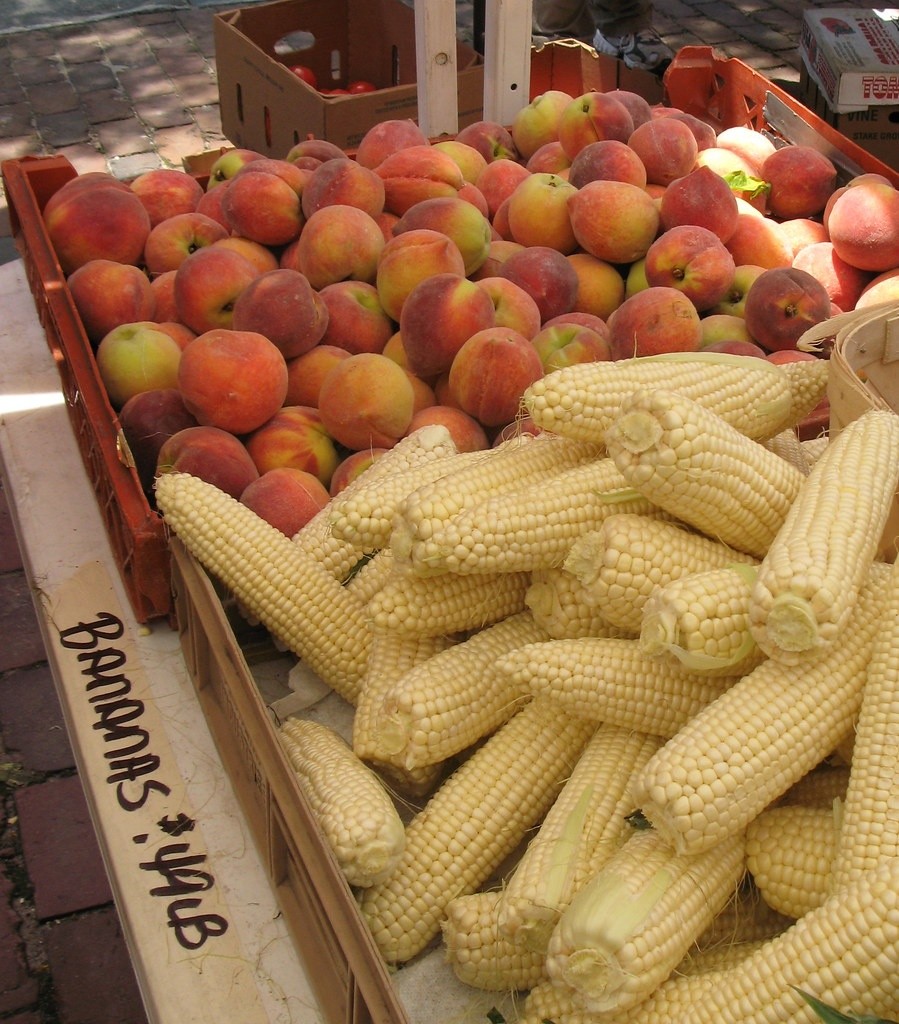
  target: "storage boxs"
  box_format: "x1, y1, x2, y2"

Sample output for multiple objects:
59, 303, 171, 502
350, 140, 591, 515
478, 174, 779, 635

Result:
199, 0, 530, 154
780, 9, 899, 171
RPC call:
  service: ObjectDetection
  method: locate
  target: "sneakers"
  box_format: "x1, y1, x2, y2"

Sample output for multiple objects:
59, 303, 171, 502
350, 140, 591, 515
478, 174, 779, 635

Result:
592, 26, 675, 76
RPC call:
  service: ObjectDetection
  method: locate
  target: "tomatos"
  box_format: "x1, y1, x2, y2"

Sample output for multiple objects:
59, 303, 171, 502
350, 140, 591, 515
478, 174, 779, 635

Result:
289, 64, 375, 95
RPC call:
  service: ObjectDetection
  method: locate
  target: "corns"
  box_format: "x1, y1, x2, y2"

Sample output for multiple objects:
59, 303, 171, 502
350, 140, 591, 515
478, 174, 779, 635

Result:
155, 349, 899, 1024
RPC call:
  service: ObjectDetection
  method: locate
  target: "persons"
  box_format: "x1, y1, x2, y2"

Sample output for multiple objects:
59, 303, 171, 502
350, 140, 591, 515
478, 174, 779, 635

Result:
533, 0, 676, 79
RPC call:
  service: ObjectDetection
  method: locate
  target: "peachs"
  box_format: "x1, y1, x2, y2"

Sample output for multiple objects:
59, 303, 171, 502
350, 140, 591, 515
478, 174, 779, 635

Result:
41, 87, 899, 540
